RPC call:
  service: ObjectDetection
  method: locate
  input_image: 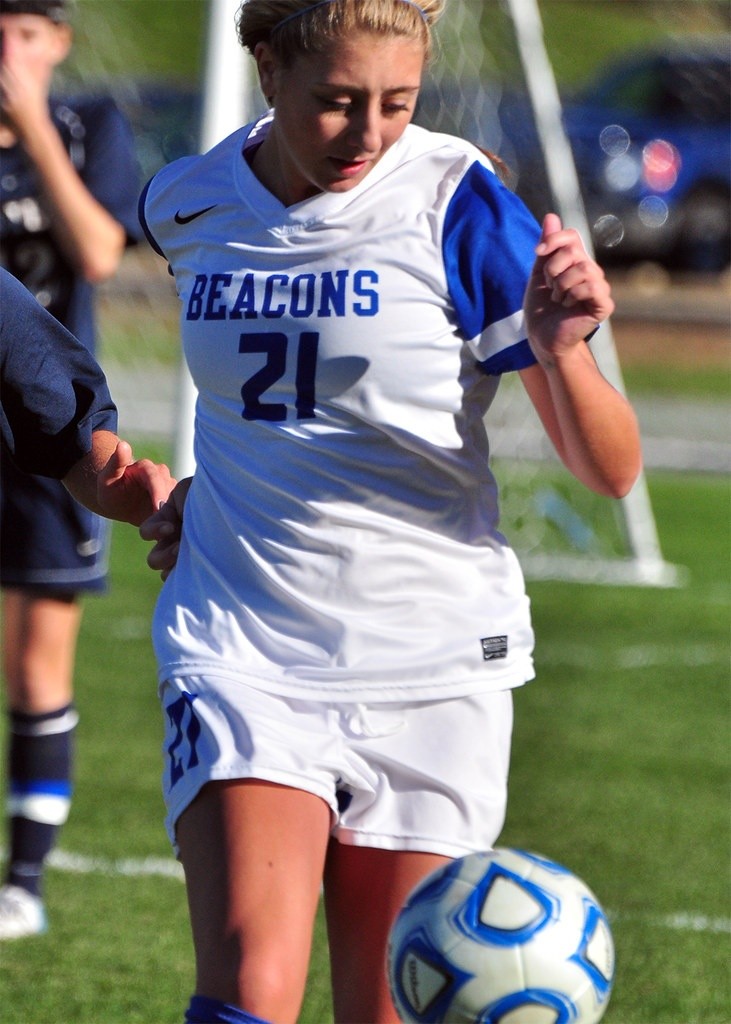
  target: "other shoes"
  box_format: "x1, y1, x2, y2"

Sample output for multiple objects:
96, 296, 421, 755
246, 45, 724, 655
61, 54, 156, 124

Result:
0, 882, 44, 941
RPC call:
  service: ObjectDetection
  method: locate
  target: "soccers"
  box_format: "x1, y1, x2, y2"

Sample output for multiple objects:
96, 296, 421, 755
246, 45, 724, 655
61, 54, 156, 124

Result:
385, 847, 617, 1024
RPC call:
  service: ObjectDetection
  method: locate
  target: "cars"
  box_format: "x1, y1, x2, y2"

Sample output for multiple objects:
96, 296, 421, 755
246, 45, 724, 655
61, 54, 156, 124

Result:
405, 42, 731, 284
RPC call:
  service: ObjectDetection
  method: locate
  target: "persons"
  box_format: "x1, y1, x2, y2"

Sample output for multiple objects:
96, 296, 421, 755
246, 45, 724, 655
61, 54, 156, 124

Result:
140, 0, 647, 1023
0, 0, 174, 940
